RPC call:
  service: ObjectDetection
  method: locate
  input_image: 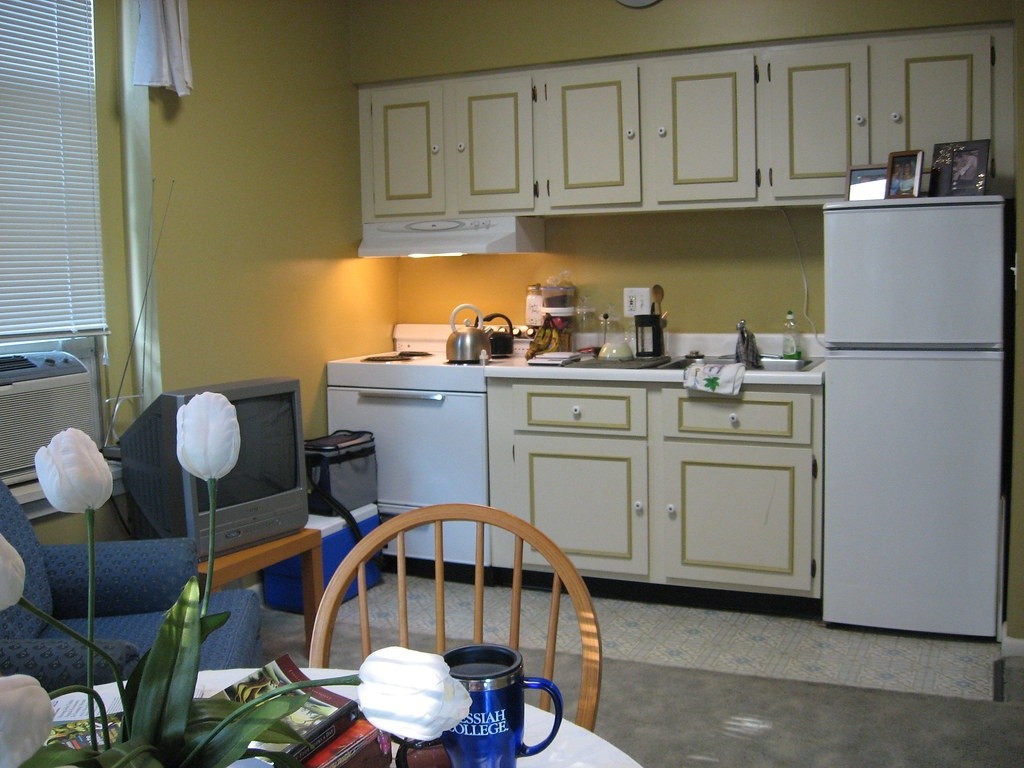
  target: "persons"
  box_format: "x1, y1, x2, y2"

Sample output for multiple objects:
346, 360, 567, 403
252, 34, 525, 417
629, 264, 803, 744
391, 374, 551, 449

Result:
890, 160, 914, 195
952, 150, 978, 181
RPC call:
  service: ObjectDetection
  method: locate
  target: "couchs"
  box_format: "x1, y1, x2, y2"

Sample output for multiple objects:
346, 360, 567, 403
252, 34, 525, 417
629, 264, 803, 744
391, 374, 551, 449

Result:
0, 477, 261, 702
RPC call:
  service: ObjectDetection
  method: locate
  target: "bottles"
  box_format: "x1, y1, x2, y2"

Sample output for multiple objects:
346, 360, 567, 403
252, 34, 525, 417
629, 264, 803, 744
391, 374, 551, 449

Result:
574, 306, 598, 350
526, 283, 545, 326
542, 307, 574, 353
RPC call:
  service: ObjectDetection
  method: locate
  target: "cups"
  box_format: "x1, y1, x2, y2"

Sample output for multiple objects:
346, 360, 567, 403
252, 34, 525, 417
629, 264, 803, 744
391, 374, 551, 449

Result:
440, 643, 564, 768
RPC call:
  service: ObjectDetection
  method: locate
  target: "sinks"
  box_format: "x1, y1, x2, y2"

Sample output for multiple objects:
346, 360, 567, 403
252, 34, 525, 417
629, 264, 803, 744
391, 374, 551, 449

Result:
657, 355, 826, 372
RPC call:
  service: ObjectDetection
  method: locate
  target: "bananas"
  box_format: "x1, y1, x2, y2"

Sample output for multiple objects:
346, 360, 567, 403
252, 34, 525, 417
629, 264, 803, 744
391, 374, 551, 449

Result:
525, 313, 559, 359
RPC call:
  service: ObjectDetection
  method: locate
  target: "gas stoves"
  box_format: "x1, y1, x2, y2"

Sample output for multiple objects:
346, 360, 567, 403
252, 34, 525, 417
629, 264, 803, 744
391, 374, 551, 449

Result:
326, 323, 505, 393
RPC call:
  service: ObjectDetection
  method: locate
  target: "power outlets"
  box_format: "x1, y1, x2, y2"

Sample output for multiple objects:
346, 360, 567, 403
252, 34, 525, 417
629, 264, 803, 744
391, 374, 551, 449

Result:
624, 287, 651, 317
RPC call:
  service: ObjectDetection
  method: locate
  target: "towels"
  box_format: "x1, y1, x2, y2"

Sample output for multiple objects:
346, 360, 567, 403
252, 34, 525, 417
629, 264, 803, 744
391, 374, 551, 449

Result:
683, 362, 747, 397
734, 330, 765, 370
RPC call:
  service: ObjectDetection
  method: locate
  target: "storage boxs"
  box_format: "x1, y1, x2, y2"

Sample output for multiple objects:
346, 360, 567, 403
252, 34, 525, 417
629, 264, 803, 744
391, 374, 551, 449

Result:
264, 503, 379, 613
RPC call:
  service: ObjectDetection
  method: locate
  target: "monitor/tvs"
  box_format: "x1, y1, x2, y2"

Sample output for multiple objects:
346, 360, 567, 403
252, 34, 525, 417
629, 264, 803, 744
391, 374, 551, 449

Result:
118, 377, 308, 563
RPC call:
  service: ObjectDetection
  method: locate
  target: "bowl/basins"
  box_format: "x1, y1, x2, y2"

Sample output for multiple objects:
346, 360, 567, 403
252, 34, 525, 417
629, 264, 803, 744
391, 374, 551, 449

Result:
597, 343, 633, 361
539, 286, 574, 308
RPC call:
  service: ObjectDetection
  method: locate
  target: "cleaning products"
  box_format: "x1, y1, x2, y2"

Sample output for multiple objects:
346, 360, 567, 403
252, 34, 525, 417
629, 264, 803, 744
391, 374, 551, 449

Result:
782, 310, 802, 359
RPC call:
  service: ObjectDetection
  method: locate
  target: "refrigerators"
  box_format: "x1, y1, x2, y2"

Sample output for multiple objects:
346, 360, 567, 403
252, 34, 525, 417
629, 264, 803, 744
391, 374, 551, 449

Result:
821, 194, 1006, 638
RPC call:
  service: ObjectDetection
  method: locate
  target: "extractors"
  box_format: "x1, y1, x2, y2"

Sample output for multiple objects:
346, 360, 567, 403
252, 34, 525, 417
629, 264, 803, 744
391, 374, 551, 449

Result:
357, 215, 546, 258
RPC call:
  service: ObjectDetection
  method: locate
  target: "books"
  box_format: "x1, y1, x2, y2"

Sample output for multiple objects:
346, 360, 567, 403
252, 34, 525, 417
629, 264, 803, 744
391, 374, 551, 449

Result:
204, 653, 391, 768
42, 709, 125, 749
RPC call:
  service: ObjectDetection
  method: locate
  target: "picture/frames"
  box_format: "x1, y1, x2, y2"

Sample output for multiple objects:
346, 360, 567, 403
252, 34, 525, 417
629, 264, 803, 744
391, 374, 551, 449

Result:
845, 165, 887, 200
927, 138, 990, 198
885, 150, 924, 199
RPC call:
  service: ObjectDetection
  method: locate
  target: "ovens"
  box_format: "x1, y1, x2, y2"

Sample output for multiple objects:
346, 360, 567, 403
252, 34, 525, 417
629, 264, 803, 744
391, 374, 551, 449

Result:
326, 386, 491, 566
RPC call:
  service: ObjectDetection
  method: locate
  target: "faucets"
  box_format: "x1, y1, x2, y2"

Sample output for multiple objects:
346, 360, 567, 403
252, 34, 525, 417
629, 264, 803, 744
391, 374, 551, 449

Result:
735, 318, 747, 345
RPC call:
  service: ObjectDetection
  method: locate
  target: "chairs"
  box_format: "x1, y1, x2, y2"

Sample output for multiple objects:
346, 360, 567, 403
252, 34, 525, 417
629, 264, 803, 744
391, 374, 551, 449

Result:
308, 504, 601, 729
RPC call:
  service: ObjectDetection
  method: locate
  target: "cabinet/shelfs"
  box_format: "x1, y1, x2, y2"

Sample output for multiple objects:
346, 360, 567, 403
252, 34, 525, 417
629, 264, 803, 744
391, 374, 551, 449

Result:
484, 378, 824, 601
351, 20, 1014, 218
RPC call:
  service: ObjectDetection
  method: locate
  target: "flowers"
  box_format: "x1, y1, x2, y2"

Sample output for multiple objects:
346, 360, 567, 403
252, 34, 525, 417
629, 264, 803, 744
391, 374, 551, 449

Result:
0, 388, 473, 768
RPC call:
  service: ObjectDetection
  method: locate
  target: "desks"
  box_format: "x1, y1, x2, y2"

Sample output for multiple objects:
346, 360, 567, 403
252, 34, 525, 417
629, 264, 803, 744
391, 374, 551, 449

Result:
49, 666, 650, 768
196, 527, 323, 659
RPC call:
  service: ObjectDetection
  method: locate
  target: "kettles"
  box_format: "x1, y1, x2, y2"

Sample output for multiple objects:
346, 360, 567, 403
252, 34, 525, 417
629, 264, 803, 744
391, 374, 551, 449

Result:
478, 312, 514, 357
444, 304, 494, 362
